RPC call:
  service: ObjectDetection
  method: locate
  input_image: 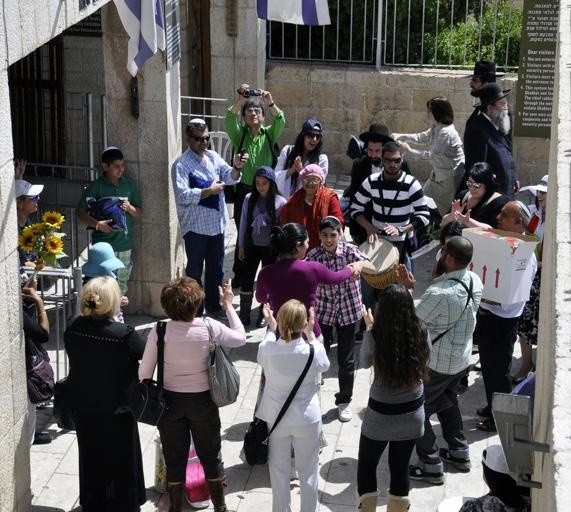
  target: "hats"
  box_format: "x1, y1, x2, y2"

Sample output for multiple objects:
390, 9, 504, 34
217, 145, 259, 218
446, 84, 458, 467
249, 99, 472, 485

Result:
472, 82, 514, 108
461, 60, 506, 80
16, 180, 45, 196
82, 241, 126, 277
255, 165, 276, 183
302, 118, 324, 133
298, 163, 326, 184
518, 175, 548, 199
482, 443, 519, 483
358, 123, 396, 145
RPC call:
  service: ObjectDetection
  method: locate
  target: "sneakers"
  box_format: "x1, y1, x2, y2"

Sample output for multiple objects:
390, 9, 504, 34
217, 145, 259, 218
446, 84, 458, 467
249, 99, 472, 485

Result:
476, 405, 496, 433
338, 402, 353, 422
439, 445, 471, 474
408, 464, 445, 486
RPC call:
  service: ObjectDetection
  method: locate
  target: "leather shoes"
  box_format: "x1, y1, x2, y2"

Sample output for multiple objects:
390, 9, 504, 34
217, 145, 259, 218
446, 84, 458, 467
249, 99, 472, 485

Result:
512, 362, 535, 386
255, 313, 267, 328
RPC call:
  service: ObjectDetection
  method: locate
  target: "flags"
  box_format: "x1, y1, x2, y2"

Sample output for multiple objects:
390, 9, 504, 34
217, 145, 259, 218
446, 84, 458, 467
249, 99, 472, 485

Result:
113, 0, 166, 78
257, 0, 331, 26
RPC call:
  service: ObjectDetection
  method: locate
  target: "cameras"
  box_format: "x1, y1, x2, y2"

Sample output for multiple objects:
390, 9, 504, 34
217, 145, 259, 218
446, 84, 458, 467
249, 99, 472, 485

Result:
243, 88, 262, 98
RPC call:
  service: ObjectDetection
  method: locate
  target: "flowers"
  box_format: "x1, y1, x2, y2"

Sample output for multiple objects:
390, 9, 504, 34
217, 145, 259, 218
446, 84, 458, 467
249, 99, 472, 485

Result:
18, 207, 69, 271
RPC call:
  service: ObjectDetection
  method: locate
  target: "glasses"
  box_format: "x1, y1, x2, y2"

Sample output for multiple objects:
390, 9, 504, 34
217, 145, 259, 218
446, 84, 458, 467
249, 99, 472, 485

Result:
380, 157, 402, 165
246, 110, 264, 114
463, 180, 482, 189
26, 194, 40, 202
306, 130, 323, 139
188, 135, 211, 143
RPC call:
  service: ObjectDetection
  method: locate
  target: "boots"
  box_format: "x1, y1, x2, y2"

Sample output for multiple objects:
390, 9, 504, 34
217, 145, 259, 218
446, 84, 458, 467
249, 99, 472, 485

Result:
207, 475, 236, 512
238, 290, 253, 326
357, 488, 381, 512
386, 487, 412, 512
167, 480, 183, 512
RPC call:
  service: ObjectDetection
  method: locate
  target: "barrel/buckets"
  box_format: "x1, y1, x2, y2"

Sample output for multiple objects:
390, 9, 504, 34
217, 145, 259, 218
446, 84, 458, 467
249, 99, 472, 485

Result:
154, 433, 169, 494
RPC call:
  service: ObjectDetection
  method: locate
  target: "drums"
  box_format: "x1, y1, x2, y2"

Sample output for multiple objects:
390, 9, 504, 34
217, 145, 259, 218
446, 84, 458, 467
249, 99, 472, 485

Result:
358, 239, 400, 291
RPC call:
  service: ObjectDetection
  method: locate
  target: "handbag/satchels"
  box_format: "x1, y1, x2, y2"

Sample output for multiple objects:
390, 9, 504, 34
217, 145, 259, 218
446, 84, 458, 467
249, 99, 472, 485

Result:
207, 348, 242, 408
53, 375, 77, 431
120, 372, 167, 428
25, 352, 57, 404
242, 418, 271, 467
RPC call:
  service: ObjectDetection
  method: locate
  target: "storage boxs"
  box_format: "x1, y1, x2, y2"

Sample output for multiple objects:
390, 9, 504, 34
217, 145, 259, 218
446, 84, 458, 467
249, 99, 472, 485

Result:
458, 224, 545, 307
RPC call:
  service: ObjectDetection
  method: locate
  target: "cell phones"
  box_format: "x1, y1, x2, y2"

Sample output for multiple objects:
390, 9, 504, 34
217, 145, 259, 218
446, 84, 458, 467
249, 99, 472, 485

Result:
240, 147, 247, 162
